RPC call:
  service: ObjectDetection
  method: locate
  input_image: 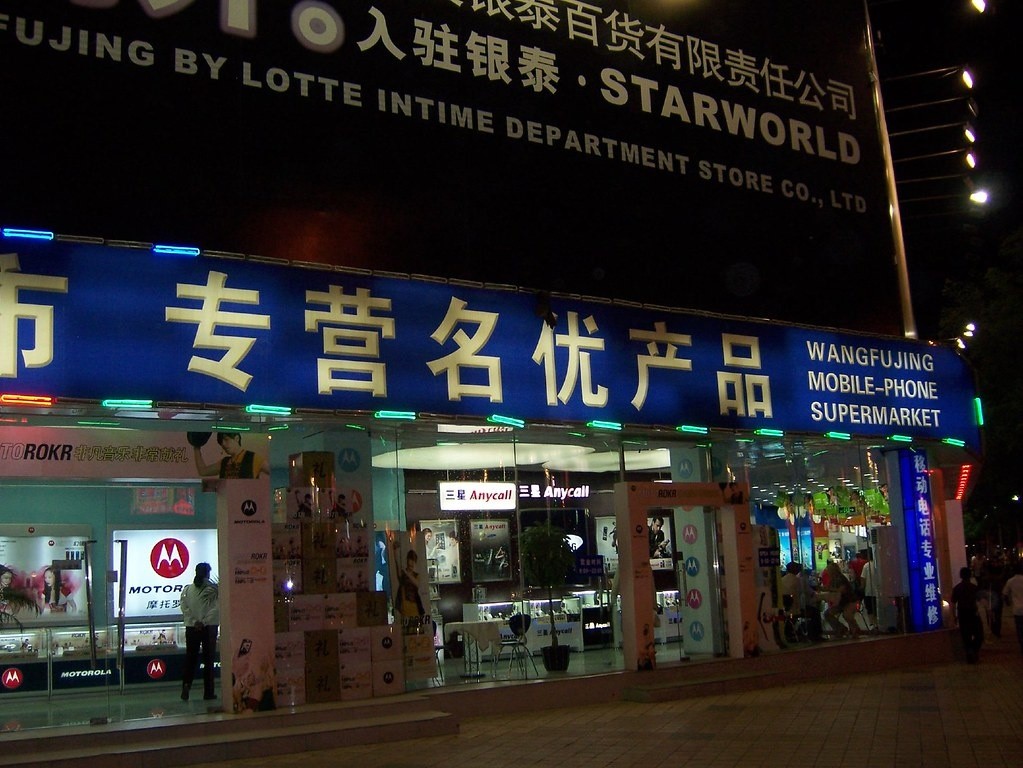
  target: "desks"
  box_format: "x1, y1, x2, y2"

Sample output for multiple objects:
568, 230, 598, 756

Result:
445, 622, 501, 678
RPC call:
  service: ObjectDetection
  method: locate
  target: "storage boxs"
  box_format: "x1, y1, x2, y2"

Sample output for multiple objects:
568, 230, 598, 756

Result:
272, 453, 440, 708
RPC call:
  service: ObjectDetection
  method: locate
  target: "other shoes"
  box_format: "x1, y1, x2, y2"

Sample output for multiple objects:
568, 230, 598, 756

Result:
852, 628, 859, 640
203, 694, 218, 700
181, 684, 189, 700
835, 626, 848, 640
812, 635, 827, 643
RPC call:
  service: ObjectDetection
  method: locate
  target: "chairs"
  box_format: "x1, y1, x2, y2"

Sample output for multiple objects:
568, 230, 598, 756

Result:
493, 615, 538, 674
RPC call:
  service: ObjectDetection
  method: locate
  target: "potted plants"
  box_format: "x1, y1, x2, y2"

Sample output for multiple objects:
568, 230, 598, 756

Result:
516, 515, 580, 671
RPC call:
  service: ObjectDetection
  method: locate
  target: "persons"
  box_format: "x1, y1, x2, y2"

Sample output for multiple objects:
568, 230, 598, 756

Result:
0, 565, 17, 616
950, 545, 1023, 665
160, 630, 167, 644
41, 567, 76, 614
25, 639, 29, 648
393, 541, 425, 634
782, 562, 829, 642
295, 490, 351, 525
187, 431, 261, 480
180, 563, 219, 700
495, 547, 510, 578
609, 522, 619, 554
446, 530, 458, 576
422, 528, 439, 559
822, 548, 877, 639
649, 515, 668, 559
609, 554, 661, 655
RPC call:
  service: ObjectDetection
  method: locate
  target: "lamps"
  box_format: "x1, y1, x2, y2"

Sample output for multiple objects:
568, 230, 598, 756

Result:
777, 441, 821, 525
